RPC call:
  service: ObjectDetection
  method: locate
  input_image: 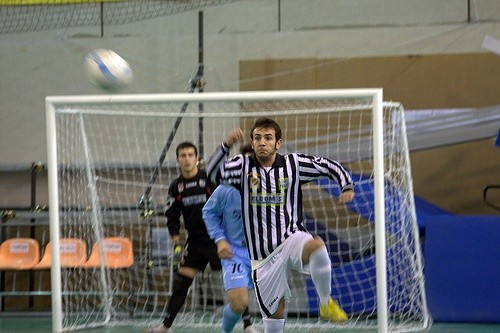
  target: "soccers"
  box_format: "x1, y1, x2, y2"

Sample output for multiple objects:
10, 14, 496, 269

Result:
85, 48, 133, 90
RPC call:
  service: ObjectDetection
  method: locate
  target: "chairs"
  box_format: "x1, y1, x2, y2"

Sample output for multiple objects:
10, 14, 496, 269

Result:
0, 238, 133, 269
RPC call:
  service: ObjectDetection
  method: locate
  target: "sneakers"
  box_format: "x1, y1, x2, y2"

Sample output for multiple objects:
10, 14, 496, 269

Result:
244, 325, 260, 333
320, 298, 349, 321
146, 323, 172, 333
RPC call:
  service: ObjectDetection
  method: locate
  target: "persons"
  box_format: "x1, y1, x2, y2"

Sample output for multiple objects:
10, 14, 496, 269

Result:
204, 118, 355, 333
202, 143, 258, 332
142, 141, 260, 333
